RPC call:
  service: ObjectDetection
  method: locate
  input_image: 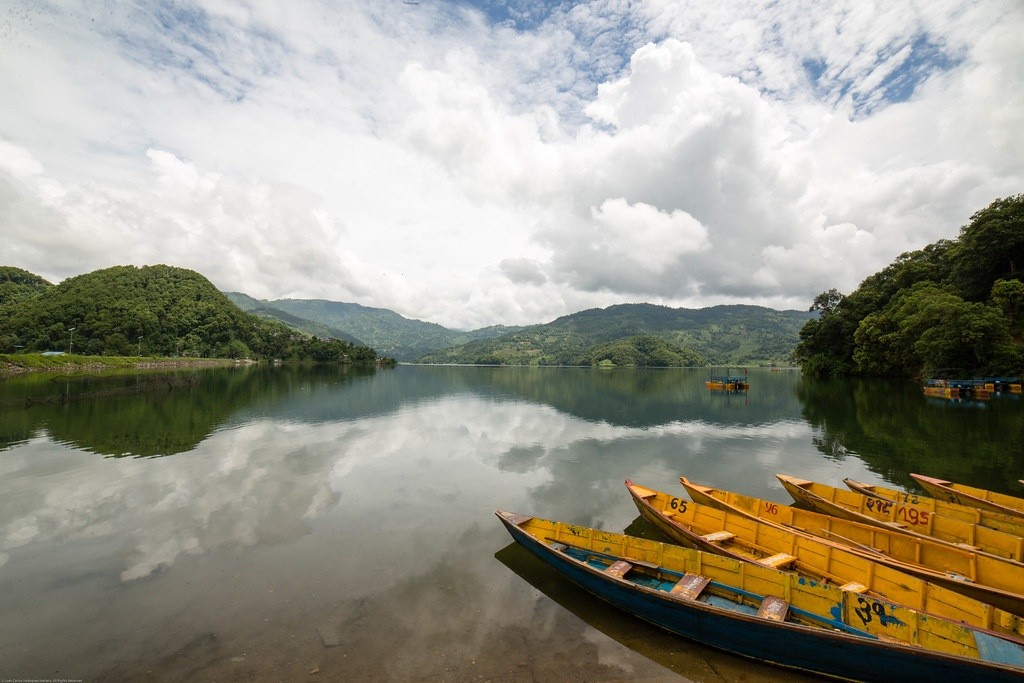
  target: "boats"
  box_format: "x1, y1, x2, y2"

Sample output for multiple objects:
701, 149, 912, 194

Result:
705, 364, 751, 393
623, 470, 1024, 642
494, 509, 1024, 683
920, 377, 963, 400
964, 376, 1022, 402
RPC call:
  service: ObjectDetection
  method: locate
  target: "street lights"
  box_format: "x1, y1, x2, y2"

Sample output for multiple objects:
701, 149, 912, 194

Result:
138, 335, 144, 357
68, 326, 75, 355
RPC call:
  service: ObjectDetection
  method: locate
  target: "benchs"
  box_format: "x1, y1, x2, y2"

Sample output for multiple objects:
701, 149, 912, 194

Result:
669, 572, 712, 600
699, 530, 737, 544
958, 542, 982, 551
884, 521, 907, 528
757, 595, 790, 621
603, 560, 634, 579
758, 552, 799, 569
838, 581, 869, 594
